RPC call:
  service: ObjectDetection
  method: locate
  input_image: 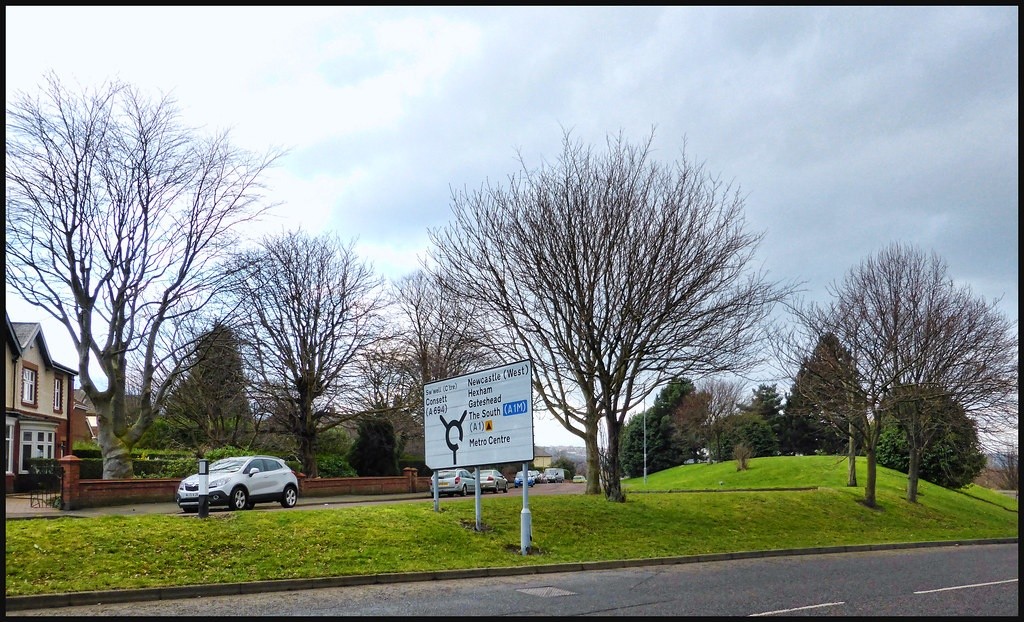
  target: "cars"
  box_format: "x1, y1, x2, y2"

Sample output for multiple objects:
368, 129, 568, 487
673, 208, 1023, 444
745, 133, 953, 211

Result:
431, 466, 481, 497
176, 454, 299, 512
471, 469, 510, 496
573, 476, 585, 484
514, 467, 564, 489
